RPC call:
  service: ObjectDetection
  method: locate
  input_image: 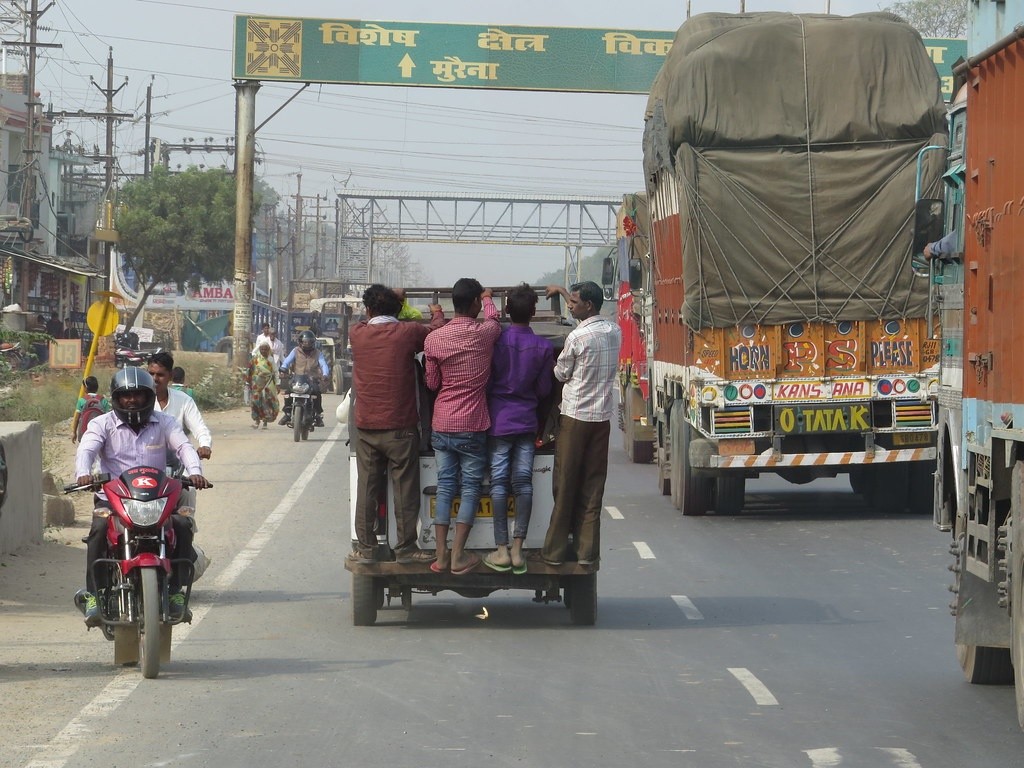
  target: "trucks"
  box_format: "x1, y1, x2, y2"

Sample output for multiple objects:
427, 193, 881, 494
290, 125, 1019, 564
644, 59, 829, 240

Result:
600, 10, 1024, 736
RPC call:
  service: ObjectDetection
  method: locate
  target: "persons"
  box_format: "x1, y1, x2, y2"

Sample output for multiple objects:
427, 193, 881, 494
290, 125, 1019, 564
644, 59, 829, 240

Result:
256, 323, 283, 370
147, 353, 212, 519
278, 330, 329, 426
31, 312, 79, 339
924, 230, 958, 261
70, 376, 111, 444
75, 367, 209, 625
527, 281, 622, 565
482, 283, 553, 574
245, 343, 281, 429
349, 284, 446, 562
424, 277, 501, 574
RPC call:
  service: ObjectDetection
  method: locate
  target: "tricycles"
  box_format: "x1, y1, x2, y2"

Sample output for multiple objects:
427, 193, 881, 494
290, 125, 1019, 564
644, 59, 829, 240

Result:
342, 285, 604, 629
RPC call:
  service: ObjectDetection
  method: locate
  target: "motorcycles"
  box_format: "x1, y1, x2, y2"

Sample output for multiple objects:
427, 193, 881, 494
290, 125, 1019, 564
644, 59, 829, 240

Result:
278, 362, 322, 442
63, 462, 214, 679
0, 341, 26, 366
113, 332, 153, 368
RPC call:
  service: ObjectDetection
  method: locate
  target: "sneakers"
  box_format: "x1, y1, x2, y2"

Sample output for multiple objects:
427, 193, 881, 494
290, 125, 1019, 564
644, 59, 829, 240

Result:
83, 590, 106, 627
168, 589, 188, 616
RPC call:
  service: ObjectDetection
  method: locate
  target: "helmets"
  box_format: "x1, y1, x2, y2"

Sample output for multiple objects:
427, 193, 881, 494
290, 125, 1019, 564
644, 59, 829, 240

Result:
110, 367, 157, 424
299, 330, 316, 351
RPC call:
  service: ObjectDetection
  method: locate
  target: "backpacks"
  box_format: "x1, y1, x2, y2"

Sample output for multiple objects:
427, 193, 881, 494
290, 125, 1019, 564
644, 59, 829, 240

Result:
76, 394, 104, 442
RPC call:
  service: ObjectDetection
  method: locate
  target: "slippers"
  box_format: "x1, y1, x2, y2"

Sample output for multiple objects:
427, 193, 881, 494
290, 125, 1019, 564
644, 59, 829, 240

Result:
430, 558, 451, 572
482, 553, 512, 571
512, 557, 528, 575
450, 554, 482, 575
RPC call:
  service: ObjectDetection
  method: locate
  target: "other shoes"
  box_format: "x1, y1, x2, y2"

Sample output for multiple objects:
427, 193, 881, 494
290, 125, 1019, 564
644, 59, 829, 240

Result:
348, 549, 374, 564
315, 417, 324, 427
252, 421, 260, 428
278, 415, 291, 425
533, 551, 562, 566
397, 549, 439, 563
577, 556, 601, 565
262, 422, 268, 429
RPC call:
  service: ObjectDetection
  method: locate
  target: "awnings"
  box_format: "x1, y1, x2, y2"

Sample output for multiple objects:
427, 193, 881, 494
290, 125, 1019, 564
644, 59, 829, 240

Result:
0, 249, 107, 279
